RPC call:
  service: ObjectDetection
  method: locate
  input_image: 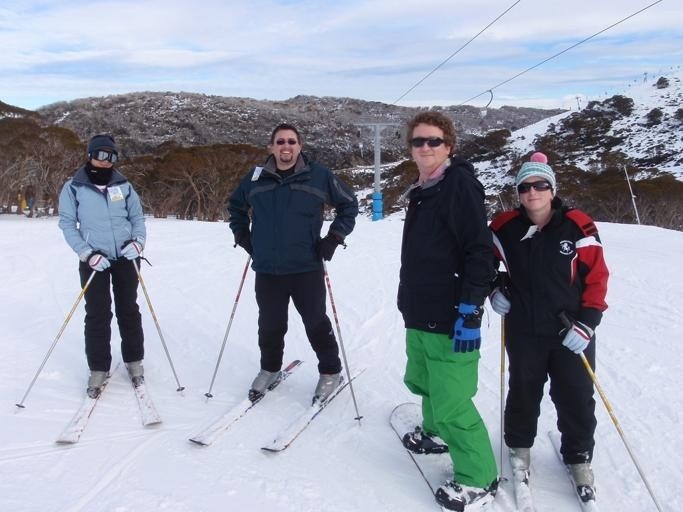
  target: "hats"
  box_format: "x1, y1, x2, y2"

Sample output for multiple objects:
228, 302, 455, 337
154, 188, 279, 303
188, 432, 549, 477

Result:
89, 135, 116, 154
516, 161, 558, 202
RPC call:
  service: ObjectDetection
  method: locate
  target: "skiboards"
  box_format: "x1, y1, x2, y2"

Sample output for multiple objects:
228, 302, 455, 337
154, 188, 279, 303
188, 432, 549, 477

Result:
56, 360, 161, 443
189, 360, 367, 451
513, 431, 596, 512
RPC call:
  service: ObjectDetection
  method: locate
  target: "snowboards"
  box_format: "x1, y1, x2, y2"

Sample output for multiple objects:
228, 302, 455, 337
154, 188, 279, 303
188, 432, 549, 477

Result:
390, 403, 495, 512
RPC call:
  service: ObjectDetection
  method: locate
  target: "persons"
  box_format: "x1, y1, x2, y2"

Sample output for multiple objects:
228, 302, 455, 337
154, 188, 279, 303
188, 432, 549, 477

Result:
394, 109, 500, 511
54, 131, 148, 401
490, 149, 611, 510
226, 123, 360, 408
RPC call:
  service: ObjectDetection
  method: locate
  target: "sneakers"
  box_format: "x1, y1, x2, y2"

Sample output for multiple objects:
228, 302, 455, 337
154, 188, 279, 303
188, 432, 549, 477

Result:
568, 463, 595, 487
508, 447, 531, 471
402, 425, 449, 455
88, 368, 111, 390
123, 358, 144, 379
314, 369, 342, 398
435, 474, 502, 512
251, 367, 282, 394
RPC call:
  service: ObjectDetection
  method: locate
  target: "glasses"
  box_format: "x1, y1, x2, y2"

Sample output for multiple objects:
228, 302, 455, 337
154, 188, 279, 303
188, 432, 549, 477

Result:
410, 136, 446, 148
276, 138, 298, 145
519, 180, 552, 193
88, 150, 117, 164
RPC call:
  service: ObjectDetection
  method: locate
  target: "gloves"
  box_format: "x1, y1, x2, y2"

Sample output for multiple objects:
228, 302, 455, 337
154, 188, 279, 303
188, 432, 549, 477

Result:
120, 240, 143, 261
448, 295, 485, 353
487, 268, 512, 318
87, 252, 112, 273
558, 306, 604, 356
235, 239, 254, 258
314, 231, 344, 261
